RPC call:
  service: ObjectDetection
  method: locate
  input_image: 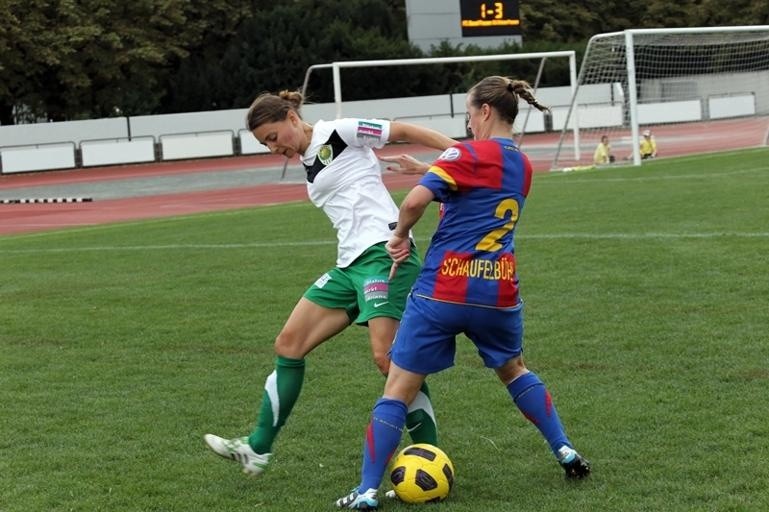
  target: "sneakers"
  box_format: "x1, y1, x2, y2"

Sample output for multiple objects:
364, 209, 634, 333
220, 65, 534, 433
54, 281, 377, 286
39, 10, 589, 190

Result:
557, 445, 590, 479
335, 487, 379, 510
203, 433, 274, 476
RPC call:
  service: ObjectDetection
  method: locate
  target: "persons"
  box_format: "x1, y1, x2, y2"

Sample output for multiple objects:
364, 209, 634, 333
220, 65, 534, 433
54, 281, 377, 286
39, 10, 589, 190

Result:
593, 136, 616, 166
622, 129, 658, 162
328, 74, 592, 512
203, 88, 461, 480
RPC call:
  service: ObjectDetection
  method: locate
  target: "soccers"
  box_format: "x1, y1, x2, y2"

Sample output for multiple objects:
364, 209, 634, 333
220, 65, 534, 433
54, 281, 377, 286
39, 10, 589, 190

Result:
392, 442, 454, 504
